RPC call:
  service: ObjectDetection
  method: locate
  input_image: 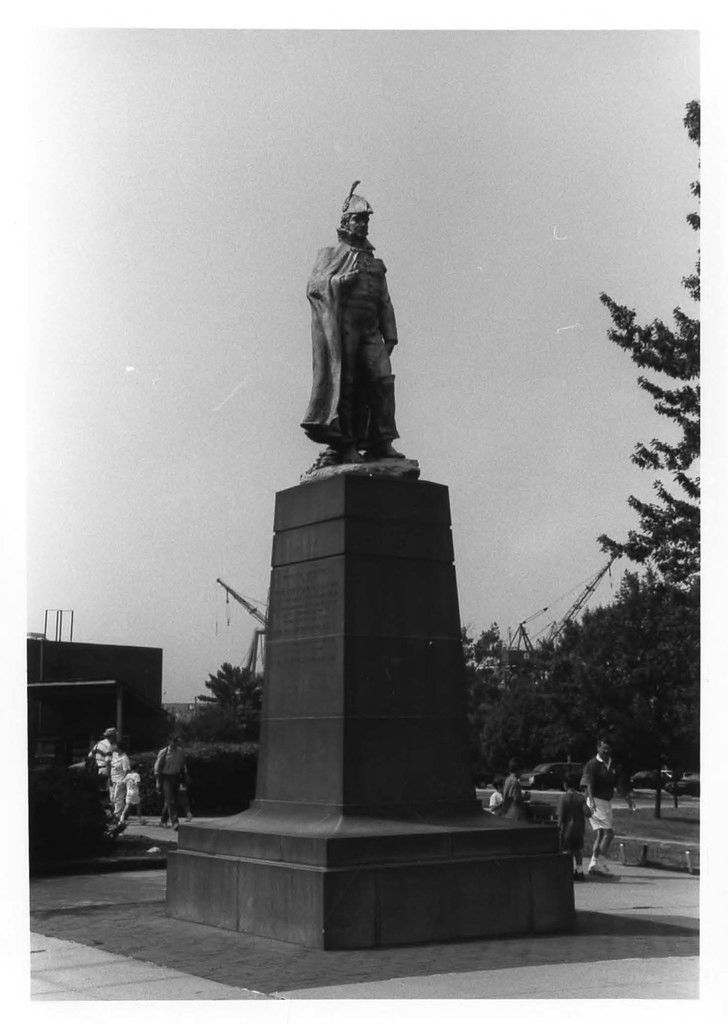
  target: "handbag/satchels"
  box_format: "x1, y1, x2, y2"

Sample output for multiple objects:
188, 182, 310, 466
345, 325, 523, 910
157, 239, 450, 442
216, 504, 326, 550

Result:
84, 757, 98, 773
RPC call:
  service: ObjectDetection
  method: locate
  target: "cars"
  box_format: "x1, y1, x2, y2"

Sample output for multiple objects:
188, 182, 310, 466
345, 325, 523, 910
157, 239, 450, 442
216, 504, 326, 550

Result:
665, 773, 700, 797
517, 762, 685, 794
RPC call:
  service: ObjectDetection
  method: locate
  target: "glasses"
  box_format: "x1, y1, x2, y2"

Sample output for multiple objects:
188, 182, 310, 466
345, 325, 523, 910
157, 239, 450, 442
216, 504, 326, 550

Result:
171, 739, 179, 742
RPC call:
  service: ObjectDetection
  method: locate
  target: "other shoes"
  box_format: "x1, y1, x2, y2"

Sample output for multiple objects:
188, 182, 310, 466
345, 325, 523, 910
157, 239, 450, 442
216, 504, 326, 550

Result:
173, 823, 178, 830
160, 821, 169, 829
573, 871, 584, 880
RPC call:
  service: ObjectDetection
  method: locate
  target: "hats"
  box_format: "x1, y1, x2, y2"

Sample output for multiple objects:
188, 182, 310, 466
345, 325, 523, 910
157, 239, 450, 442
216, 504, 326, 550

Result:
130, 761, 143, 768
103, 728, 119, 736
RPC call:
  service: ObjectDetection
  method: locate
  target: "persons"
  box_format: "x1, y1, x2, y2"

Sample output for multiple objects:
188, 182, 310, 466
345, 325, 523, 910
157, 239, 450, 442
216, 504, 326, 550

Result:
88, 727, 147, 827
300, 180, 406, 466
555, 777, 595, 881
489, 757, 526, 820
580, 737, 639, 878
154, 735, 193, 830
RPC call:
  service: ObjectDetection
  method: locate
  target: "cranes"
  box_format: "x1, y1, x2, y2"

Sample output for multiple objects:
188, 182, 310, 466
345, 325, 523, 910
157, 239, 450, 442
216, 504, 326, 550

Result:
216, 578, 266, 629
500, 552, 621, 666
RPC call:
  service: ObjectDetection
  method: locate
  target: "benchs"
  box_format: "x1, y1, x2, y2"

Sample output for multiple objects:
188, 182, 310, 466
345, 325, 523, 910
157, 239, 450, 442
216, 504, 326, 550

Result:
613, 835, 699, 875
